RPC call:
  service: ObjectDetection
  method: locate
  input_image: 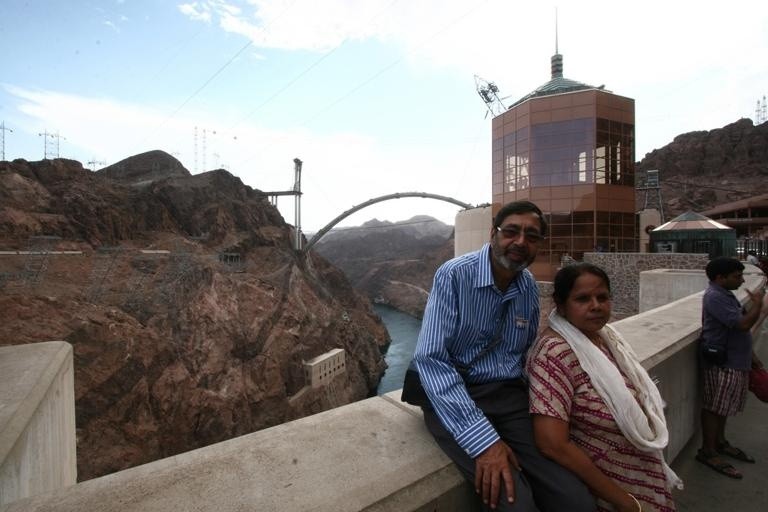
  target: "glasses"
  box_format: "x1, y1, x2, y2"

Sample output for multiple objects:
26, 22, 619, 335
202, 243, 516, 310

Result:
497, 226, 545, 242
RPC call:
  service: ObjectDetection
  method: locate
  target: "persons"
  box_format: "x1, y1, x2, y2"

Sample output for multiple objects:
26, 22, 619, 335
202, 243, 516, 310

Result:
745, 249, 764, 266
411, 199, 598, 512
697, 257, 765, 481
524, 263, 684, 512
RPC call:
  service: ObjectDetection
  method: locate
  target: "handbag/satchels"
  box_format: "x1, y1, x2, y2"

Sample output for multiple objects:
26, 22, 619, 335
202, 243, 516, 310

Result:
747, 368, 768, 402
401, 361, 469, 411
698, 343, 727, 366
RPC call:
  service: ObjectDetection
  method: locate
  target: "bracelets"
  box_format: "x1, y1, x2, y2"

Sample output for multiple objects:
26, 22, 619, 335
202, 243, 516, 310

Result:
626, 491, 649, 510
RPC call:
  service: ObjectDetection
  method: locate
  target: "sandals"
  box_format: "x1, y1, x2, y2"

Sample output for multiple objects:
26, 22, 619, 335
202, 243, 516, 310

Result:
717, 441, 754, 462
697, 449, 742, 478
